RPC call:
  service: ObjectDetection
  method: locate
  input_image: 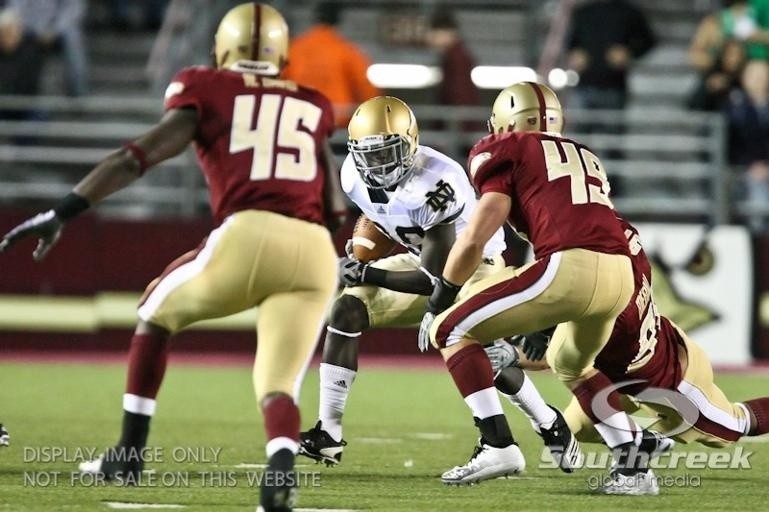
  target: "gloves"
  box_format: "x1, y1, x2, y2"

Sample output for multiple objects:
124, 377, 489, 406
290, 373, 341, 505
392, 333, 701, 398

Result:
1, 190, 90, 261
522, 327, 554, 362
483, 337, 520, 373
417, 276, 460, 353
339, 254, 384, 289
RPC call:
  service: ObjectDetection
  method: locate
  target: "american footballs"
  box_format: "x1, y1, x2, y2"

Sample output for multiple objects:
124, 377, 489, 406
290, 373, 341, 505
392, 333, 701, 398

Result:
352, 212, 397, 263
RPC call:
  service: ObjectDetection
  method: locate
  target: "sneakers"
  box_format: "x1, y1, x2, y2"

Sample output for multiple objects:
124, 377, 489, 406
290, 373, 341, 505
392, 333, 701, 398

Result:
257, 473, 297, 511
440, 437, 525, 486
531, 405, 585, 472
297, 421, 345, 466
596, 468, 658, 496
77, 445, 143, 483
635, 429, 672, 459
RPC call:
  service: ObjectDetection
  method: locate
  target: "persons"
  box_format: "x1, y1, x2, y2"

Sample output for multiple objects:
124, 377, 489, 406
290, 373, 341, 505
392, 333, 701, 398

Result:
1, 1, 347, 511
297, 93, 581, 476
416, 79, 660, 499
483, 313, 769, 461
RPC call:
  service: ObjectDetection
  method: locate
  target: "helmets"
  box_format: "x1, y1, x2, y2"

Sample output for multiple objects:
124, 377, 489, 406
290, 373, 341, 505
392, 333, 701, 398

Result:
486, 81, 563, 134
215, 3, 290, 76
347, 95, 418, 191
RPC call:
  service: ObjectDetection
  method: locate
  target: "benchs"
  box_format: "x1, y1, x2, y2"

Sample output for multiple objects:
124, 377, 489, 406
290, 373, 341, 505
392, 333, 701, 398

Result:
0, 1, 769, 227
